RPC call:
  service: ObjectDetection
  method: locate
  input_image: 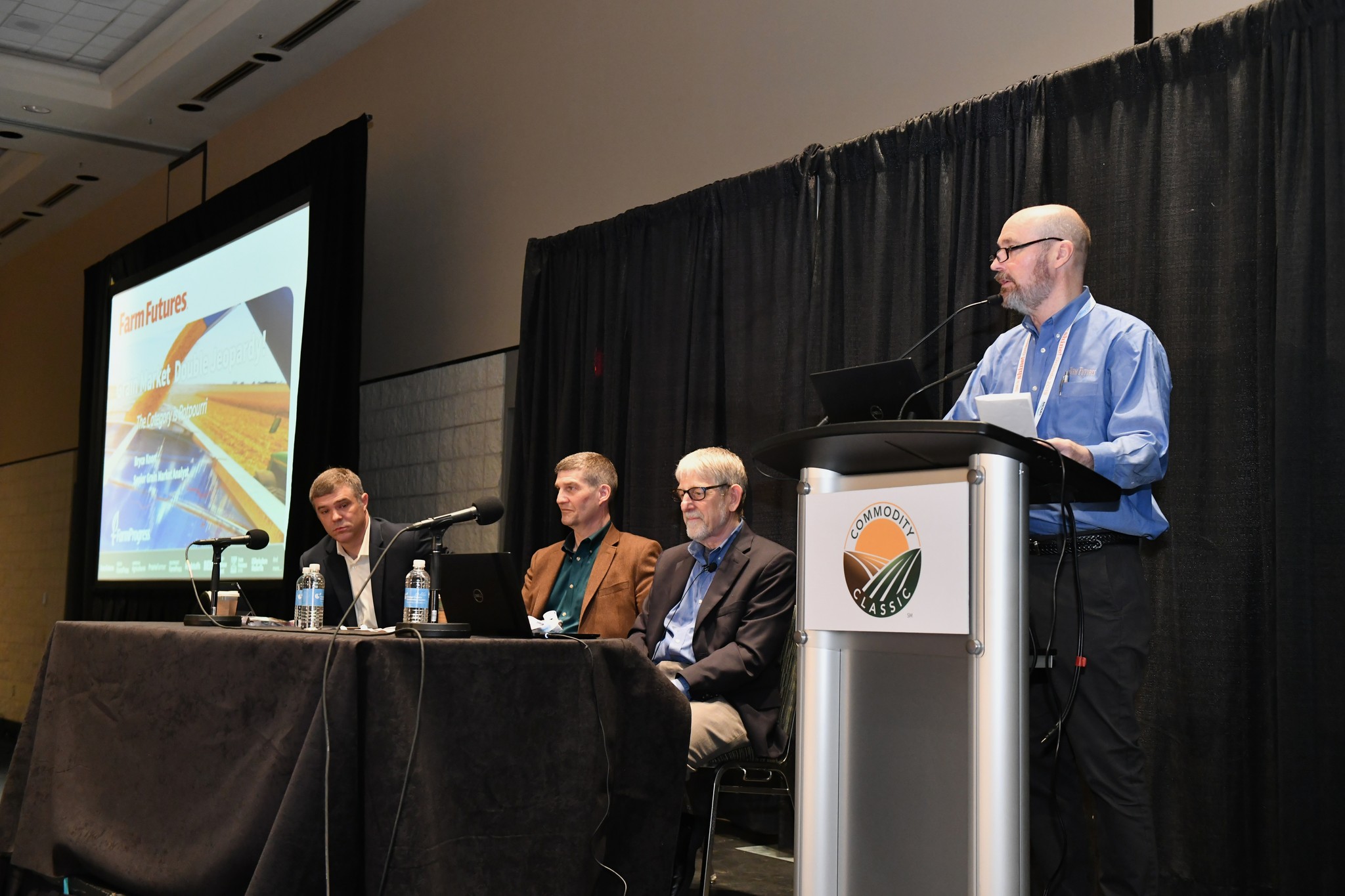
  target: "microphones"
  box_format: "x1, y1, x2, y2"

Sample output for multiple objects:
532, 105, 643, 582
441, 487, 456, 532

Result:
193, 529, 269, 550
815, 294, 1003, 426
406, 495, 504, 531
896, 362, 978, 421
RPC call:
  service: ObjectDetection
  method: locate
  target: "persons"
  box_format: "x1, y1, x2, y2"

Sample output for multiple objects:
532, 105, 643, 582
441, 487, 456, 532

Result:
627, 445, 793, 780
944, 205, 1174, 896
300, 468, 455, 629
522, 452, 663, 639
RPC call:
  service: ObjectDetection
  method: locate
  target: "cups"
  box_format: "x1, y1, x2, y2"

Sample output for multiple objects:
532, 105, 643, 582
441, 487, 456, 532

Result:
216, 591, 240, 616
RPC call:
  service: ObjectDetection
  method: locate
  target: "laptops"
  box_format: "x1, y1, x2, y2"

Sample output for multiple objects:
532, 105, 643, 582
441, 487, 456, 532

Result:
809, 357, 935, 422
431, 551, 600, 639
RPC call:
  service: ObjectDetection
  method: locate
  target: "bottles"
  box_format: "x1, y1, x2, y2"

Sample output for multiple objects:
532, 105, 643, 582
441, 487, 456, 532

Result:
301, 564, 325, 631
294, 567, 310, 627
402, 560, 430, 623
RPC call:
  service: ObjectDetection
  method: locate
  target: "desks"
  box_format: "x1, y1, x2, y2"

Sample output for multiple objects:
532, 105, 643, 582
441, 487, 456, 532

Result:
0, 621, 689, 896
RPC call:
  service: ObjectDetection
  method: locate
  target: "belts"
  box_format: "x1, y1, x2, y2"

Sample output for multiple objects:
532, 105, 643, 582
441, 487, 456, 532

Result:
1028, 531, 1140, 556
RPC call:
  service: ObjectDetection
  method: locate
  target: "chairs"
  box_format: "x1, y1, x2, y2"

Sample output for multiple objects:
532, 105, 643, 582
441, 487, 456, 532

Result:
696, 605, 796, 896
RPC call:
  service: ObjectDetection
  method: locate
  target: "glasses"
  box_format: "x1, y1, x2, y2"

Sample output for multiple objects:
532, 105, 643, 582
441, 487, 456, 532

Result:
988, 236, 1065, 264
671, 483, 732, 503
